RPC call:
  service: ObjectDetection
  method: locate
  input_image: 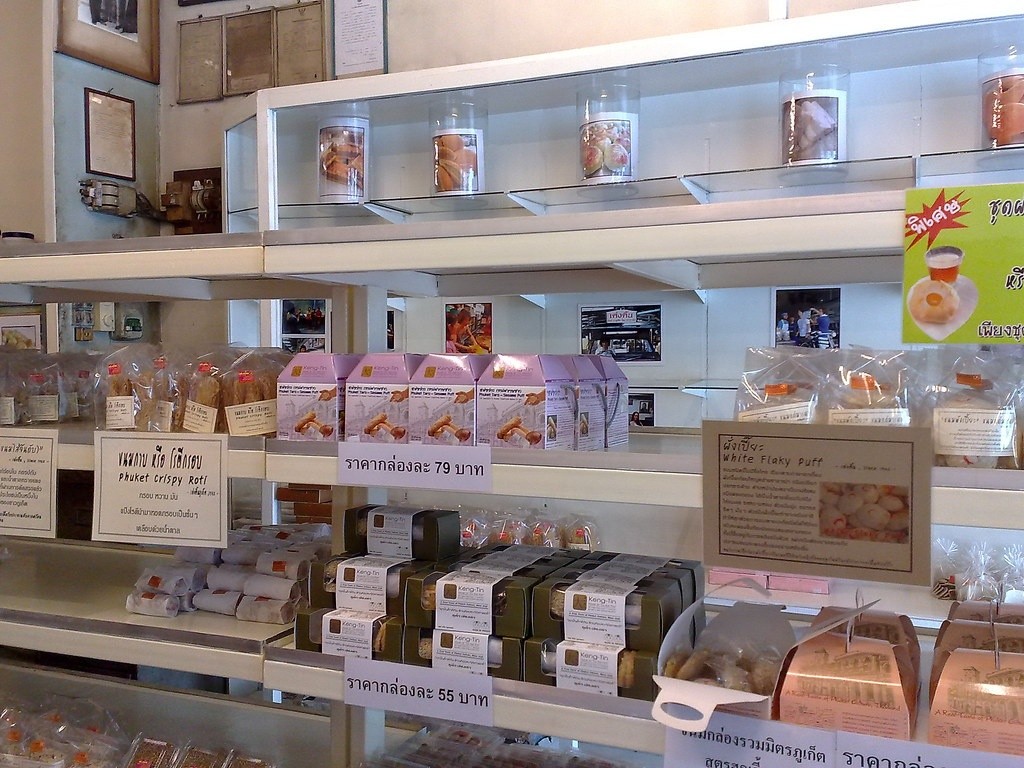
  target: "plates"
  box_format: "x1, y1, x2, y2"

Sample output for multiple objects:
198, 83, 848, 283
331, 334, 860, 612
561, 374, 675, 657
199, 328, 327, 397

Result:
907, 274, 977, 341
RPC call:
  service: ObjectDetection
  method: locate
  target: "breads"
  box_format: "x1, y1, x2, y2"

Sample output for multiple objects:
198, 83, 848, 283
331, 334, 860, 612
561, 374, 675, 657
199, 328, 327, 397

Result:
104, 365, 281, 439
666, 649, 781, 696
781, 101, 836, 155
983, 76, 1024, 145
907, 279, 960, 324
320, 130, 365, 191
434, 133, 477, 190
0, 704, 274, 768
460, 520, 601, 552
579, 120, 631, 176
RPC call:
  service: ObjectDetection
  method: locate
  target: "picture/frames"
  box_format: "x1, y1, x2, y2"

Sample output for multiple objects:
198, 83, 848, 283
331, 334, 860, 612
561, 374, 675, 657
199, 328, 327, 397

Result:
54, 0, 161, 85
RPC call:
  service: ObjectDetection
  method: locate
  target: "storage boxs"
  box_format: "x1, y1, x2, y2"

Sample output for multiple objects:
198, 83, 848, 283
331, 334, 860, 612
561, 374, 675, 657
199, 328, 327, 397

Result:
289, 506, 1024, 757
279, 355, 629, 458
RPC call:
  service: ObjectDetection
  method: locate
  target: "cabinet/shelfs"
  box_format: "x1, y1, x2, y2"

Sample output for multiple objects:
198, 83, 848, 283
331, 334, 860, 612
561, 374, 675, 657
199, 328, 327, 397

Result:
0, 233, 353, 768
222, 0, 1024, 234
263, 182, 1024, 768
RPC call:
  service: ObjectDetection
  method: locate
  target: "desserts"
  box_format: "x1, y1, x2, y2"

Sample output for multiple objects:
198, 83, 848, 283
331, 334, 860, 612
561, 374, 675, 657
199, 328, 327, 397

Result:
817, 483, 909, 545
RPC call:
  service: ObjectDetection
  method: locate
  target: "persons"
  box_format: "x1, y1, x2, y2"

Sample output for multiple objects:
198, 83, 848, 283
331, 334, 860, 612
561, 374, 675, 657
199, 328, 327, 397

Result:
89, 0, 137, 34
594, 337, 616, 357
299, 345, 306, 352
776, 305, 830, 350
446, 309, 478, 354
286, 306, 322, 334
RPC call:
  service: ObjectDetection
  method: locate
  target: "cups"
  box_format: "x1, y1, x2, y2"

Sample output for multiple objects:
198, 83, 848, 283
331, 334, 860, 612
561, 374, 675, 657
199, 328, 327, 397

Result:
575, 69, 638, 183
428, 91, 486, 196
924, 244, 967, 284
314, 101, 369, 205
977, 45, 1023, 151
778, 63, 851, 167
